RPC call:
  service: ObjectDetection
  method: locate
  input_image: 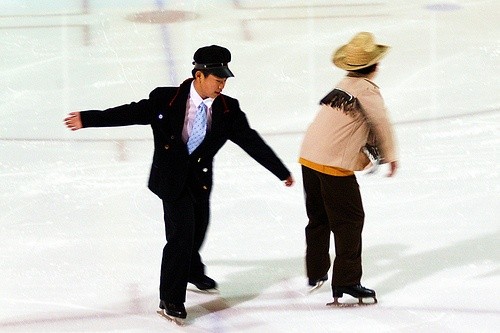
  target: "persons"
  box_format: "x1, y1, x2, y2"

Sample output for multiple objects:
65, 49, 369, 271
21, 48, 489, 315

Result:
63, 45, 294, 326
297, 32, 399, 305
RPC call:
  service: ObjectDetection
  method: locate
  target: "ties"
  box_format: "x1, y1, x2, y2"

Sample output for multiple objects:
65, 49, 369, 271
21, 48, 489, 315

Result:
187, 102, 207, 155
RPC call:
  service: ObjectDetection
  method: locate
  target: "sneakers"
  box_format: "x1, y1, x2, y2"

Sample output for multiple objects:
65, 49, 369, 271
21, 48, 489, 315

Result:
307, 273, 329, 292
326, 284, 377, 306
157, 299, 187, 326
186, 275, 221, 294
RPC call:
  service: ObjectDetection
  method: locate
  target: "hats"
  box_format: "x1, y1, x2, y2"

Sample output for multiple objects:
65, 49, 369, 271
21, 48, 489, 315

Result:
192, 44, 235, 77
333, 32, 392, 70
